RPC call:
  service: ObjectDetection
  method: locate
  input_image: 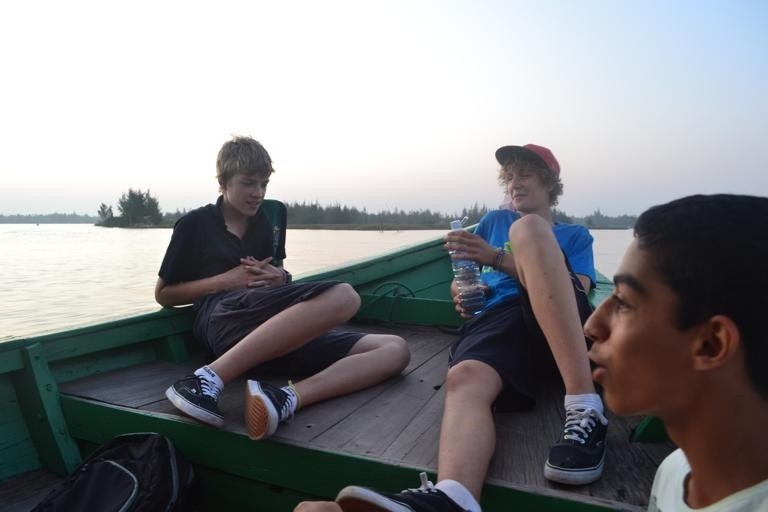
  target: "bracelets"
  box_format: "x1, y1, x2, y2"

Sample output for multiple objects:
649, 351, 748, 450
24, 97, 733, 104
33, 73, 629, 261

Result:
494, 247, 505, 270
281, 268, 292, 286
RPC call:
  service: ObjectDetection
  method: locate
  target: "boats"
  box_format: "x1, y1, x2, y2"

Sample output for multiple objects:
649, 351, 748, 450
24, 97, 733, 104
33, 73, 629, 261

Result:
0, 216, 679, 512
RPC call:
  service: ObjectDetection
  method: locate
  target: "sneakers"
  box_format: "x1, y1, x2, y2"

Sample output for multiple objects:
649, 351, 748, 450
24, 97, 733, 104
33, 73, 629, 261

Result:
165, 373, 225, 429
543, 407, 609, 486
244, 379, 294, 441
334, 471, 466, 512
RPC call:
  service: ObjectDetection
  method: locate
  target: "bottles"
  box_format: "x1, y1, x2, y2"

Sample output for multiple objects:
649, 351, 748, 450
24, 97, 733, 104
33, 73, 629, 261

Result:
446, 216, 487, 314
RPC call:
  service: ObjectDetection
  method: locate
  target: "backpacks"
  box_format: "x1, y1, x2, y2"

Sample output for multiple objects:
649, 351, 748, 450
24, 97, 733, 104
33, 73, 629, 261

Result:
30, 430, 194, 511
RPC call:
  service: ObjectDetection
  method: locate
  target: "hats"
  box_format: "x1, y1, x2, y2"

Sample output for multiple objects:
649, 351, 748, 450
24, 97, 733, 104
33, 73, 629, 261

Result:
495, 144, 559, 179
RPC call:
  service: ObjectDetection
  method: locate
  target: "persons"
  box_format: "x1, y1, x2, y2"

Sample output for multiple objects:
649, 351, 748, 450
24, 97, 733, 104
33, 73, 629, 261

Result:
154, 134, 412, 442
334, 141, 611, 512
581, 193, 768, 512
